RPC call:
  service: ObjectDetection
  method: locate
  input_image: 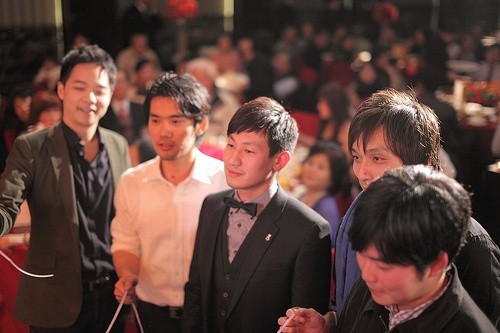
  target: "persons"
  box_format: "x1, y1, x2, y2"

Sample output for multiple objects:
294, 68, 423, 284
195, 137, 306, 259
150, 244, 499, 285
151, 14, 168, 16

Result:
107, 69, 231, 331
333, 166, 498, 333
180, 97, 331, 333
4, 14, 500, 232
278, 87, 496, 333
0, 45, 135, 331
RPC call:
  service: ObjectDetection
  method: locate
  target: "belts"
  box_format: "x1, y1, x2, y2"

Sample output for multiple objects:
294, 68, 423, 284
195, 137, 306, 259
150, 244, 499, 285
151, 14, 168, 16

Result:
83, 273, 116, 292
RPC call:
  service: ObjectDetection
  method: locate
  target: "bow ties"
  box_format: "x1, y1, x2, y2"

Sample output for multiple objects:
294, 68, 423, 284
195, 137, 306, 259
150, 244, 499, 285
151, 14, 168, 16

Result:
222, 197, 258, 217
